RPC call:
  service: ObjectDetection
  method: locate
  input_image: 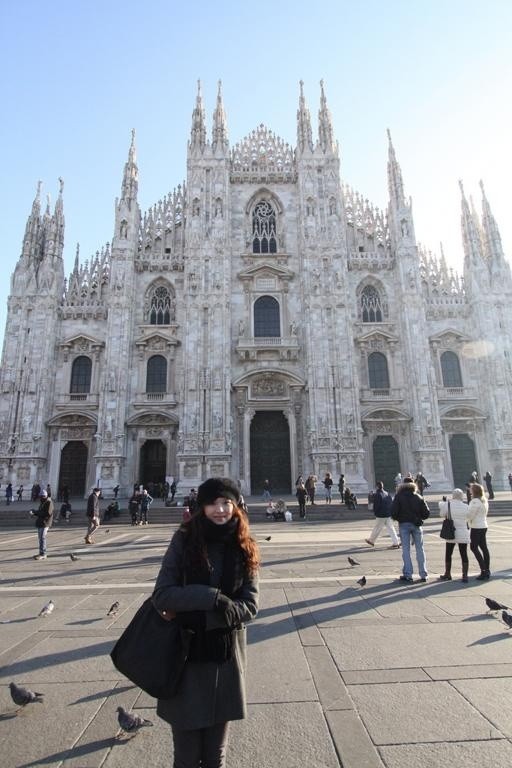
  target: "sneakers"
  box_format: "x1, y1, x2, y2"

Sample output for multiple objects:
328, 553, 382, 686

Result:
84, 536, 97, 544
34, 554, 47, 560
399, 576, 430, 583
364, 538, 400, 550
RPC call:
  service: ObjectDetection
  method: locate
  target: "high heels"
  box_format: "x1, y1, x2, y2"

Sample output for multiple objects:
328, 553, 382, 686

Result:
475, 570, 491, 581
439, 573, 469, 583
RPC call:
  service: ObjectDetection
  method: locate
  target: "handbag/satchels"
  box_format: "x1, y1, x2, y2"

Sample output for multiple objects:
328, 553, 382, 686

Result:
440, 518, 455, 540
108, 597, 196, 701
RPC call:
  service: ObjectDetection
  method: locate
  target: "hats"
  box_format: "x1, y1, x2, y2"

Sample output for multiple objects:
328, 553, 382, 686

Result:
39, 489, 47, 496
198, 477, 240, 505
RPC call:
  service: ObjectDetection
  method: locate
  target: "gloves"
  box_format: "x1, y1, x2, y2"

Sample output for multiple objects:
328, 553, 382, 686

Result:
166, 613, 205, 640
212, 591, 242, 629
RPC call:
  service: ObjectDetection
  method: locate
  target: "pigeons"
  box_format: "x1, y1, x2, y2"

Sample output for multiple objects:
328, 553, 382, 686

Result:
71, 553, 81, 561
8, 682, 45, 713
486, 598, 508, 610
348, 557, 360, 566
38, 600, 55, 618
357, 576, 366, 587
502, 611, 512, 629
107, 602, 120, 616
265, 536, 271, 541
106, 529, 109, 533
115, 706, 153, 737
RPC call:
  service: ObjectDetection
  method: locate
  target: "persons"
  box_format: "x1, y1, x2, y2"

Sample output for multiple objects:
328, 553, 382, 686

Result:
84, 488, 101, 544
471, 470, 512, 500
438, 488, 469, 583
102, 481, 197, 525
364, 481, 398, 548
394, 471, 429, 495
6, 483, 51, 505
29, 489, 54, 560
151, 477, 260, 768
237, 473, 374, 521
55, 483, 72, 523
390, 477, 430, 583
465, 484, 491, 580
